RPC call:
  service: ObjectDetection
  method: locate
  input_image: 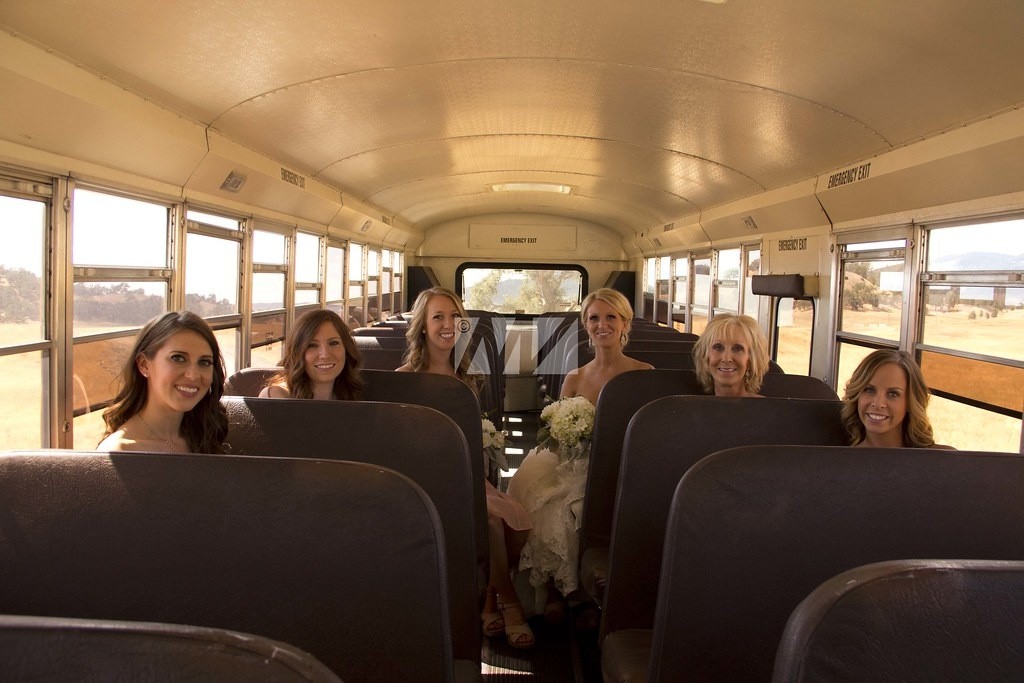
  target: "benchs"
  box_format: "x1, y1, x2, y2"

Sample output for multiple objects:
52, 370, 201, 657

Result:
537, 312, 1024, 683
0, 310, 506, 683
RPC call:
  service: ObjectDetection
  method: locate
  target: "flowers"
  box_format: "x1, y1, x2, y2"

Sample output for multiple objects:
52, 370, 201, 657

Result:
482, 414, 514, 472
536, 395, 595, 481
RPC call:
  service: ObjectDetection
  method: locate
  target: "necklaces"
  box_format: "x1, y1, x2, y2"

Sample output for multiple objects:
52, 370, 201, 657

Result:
136, 412, 177, 453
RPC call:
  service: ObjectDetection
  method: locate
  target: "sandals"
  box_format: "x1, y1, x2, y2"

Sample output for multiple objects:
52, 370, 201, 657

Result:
480, 592, 505, 638
499, 599, 536, 648
545, 598, 565, 627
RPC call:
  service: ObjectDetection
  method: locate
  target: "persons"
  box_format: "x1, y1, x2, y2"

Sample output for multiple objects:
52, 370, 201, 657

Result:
258, 309, 366, 402
841, 349, 958, 450
95, 309, 229, 456
691, 313, 770, 398
506, 287, 656, 621
395, 286, 536, 649
